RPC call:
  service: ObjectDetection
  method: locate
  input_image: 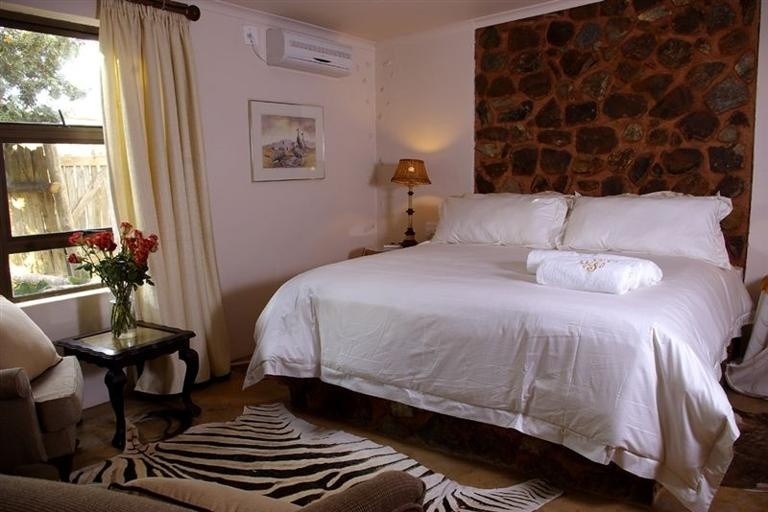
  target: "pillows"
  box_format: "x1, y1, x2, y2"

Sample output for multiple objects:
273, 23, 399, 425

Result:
563, 191, 732, 272
0, 292, 60, 384
431, 195, 567, 248
107, 478, 301, 512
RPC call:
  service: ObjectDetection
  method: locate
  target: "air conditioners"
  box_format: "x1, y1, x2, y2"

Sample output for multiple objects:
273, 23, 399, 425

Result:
266, 26, 353, 77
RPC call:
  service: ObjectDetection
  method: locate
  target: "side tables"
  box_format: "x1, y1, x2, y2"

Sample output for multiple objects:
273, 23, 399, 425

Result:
53, 320, 203, 450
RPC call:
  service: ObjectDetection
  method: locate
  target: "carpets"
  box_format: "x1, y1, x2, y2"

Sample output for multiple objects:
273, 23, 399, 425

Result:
66, 402, 560, 512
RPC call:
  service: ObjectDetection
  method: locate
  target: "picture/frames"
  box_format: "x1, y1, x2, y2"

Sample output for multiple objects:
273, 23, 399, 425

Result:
247, 99, 327, 184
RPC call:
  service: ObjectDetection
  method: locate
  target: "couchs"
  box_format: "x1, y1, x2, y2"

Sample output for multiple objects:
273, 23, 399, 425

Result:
0, 356, 80, 477
0, 471, 426, 512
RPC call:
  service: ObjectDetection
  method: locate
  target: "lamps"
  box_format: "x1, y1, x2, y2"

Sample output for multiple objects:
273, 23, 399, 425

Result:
391, 159, 430, 248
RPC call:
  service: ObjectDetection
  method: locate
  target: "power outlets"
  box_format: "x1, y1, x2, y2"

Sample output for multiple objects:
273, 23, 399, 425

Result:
243, 25, 258, 46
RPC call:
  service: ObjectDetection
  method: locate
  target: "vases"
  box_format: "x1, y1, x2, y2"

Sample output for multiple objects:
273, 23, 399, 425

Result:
108, 297, 137, 339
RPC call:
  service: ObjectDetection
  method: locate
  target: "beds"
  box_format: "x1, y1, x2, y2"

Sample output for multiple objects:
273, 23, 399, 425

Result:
277, 191, 732, 502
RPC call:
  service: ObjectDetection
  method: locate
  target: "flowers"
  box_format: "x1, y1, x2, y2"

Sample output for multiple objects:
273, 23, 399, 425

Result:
67, 222, 158, 337
